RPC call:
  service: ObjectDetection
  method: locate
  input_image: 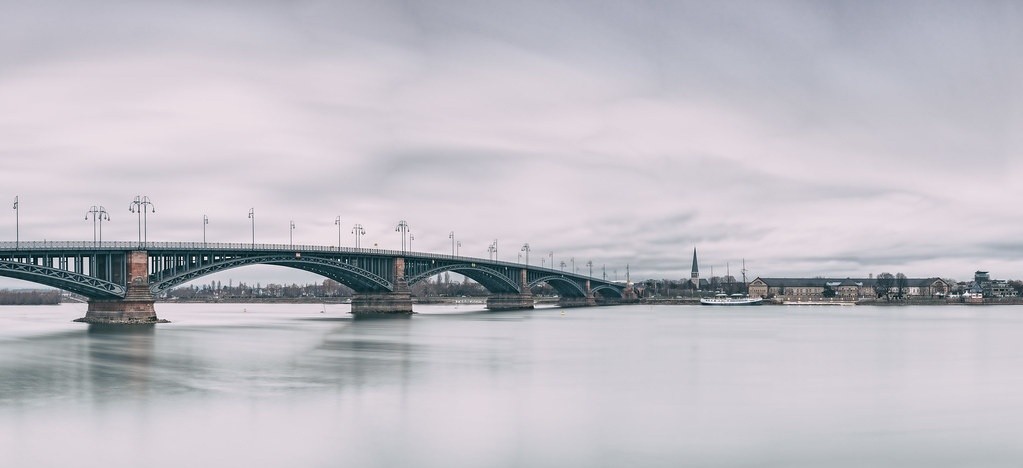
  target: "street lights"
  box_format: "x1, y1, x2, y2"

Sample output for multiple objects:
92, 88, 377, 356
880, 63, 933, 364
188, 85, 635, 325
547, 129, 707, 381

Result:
395, 218, 414, 256
13, 196, 19, 250
358, 223, 366, 249
518, 243, 531, 267
203, 214, 209, 244
488, 238, 498, 265
99, 206, 110, 246
248, 207, 255, 250
560, 256, 606, 281
289, 219, 296, 250
448, 230, 454, 259
84, 206, 97, 247
128, 195, 141, 248
334, 215, 340, 252
548, 251, 554, 270
351, 223, 358, 251
541, 258, 546, 268
142, 195, 155, 247
456, 240, 462, 260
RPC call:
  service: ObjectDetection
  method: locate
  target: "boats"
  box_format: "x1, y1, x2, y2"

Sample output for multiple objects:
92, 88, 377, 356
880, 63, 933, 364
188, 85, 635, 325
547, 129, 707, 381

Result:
700, 295, 764, 306
